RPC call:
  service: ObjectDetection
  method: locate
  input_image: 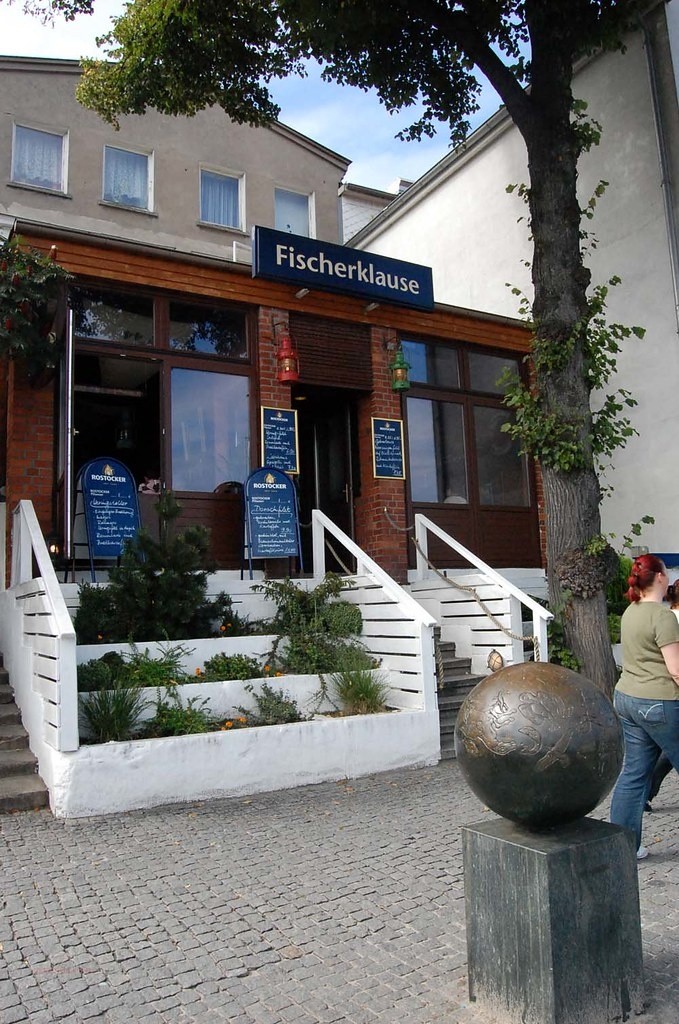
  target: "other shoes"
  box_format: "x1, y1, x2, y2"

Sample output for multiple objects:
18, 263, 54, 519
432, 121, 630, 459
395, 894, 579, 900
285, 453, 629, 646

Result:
644, 802, 653, 811
636, 844, 650, 860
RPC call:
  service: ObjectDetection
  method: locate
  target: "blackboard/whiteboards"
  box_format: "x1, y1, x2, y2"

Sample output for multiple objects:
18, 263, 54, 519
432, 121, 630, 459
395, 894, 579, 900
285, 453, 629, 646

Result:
371, 417, 406, 480
261, 406, 300, 474
244, 466, 301, 557
81, 456, 145, 558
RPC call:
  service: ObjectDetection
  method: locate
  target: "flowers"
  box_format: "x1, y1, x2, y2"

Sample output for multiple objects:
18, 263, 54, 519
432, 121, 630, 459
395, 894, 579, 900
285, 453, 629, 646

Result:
0, 233, 79, 359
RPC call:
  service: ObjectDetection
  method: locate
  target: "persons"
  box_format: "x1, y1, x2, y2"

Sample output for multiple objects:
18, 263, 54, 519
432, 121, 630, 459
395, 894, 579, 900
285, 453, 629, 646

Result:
444, 486, 467, 504
609, 555, 679, 858
138, 471, 161, 494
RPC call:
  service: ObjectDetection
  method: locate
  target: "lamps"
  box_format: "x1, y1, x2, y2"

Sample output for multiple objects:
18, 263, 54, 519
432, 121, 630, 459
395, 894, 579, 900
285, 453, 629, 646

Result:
382, 335, 413, 392
263, 315, 302, 386
114, 409, 139, 451
